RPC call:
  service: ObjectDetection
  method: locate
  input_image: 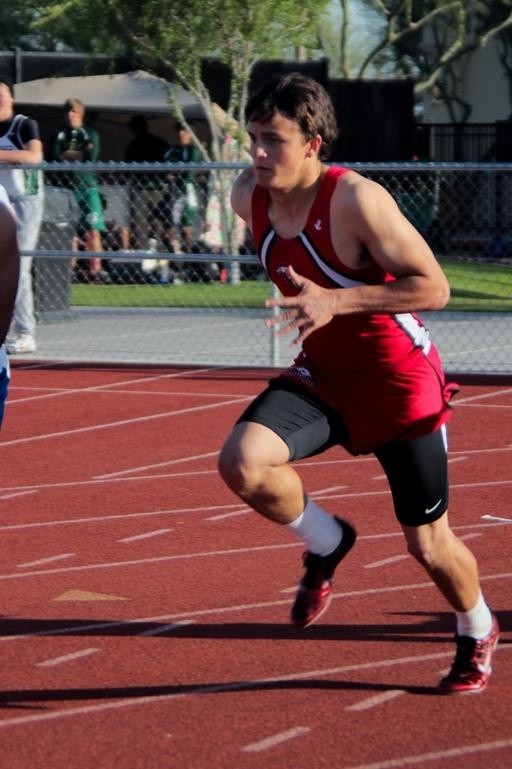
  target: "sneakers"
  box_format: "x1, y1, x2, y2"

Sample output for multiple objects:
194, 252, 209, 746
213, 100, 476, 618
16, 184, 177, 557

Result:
288, 514, 359, 631
434, 609, 502, 696
5, 332, 39, 354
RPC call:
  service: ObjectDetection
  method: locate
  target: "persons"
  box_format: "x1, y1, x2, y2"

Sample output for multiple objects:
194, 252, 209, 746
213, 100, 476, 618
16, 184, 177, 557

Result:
2, 200, 20, 431
161, 119, 208, 271
1, 82, 46, 355
119, 113, 170, 188
217, 72, 505, 697
54, 97, 108, 285
